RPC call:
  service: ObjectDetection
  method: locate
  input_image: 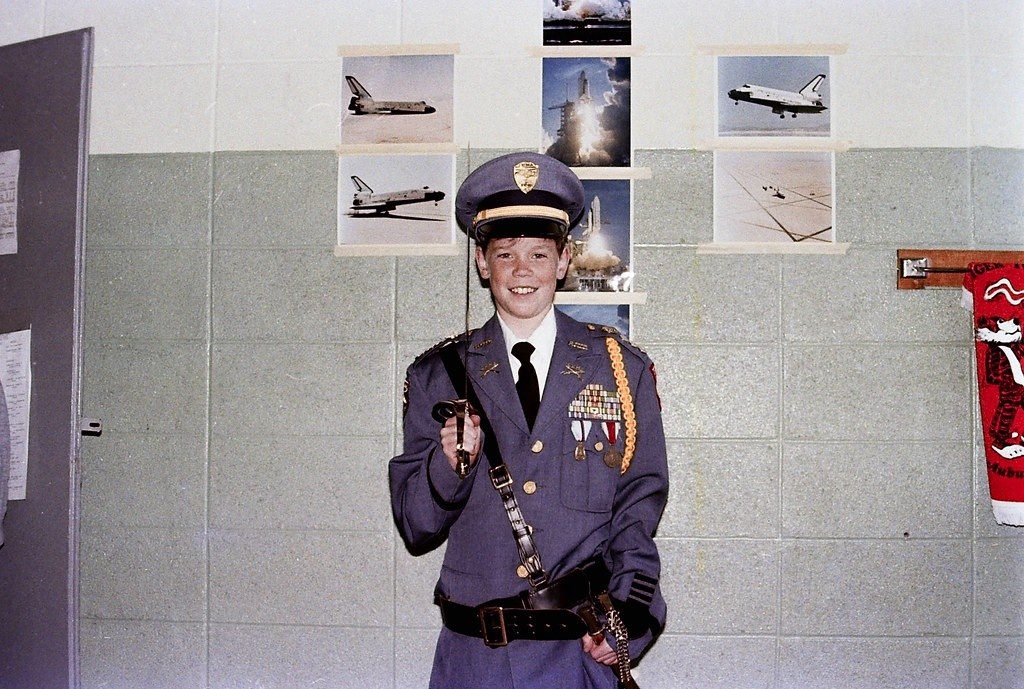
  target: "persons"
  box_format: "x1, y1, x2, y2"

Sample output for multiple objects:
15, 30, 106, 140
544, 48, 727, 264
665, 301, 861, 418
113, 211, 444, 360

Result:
387, 150, 669, 689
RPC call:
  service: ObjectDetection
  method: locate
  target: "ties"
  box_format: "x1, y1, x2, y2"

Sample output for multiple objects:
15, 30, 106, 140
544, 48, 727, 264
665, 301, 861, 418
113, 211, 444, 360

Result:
511, 342, 540, 435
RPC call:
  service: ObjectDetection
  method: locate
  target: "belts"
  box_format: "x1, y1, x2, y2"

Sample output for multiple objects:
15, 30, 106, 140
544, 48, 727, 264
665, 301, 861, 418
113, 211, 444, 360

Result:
430, 553, 609, 649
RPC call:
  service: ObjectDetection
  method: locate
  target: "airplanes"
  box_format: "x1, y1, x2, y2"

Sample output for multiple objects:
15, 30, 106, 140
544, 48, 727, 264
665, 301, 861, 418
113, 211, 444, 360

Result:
344, 75, 435, 115
349, 173, 446, 216
726, 72, 827, 120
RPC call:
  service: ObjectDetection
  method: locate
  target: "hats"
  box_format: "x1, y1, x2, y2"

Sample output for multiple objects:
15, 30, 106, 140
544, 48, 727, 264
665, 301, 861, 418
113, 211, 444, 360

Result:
455, 151, 587, 248
